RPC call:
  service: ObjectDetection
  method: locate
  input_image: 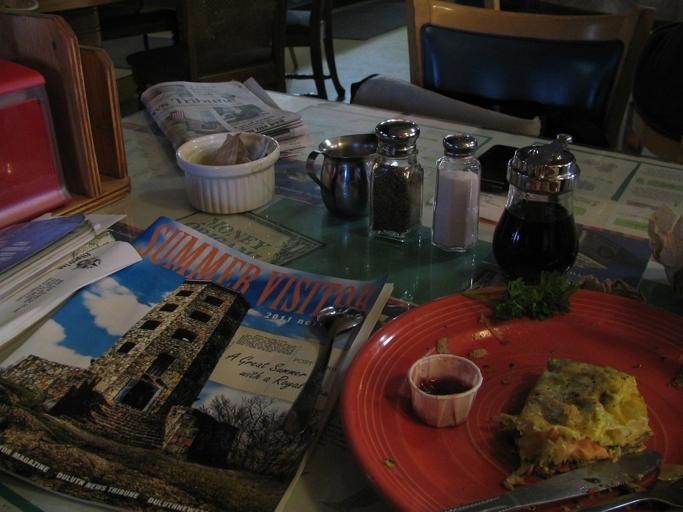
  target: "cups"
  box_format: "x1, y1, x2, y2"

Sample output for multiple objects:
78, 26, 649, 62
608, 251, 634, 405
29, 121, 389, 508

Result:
305, 133, 378, 218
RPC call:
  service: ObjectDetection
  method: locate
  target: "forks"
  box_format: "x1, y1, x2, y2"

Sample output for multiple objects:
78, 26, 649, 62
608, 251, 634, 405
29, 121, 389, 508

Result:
564, 475, 683, 512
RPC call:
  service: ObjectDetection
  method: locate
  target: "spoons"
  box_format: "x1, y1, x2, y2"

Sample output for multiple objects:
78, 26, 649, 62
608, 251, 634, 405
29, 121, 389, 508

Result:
340, 282, 683, 512
283, 304, 366, 435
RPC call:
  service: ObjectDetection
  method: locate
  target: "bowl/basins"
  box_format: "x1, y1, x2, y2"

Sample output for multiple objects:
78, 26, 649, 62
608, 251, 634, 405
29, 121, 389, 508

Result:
177, 131, 281, 214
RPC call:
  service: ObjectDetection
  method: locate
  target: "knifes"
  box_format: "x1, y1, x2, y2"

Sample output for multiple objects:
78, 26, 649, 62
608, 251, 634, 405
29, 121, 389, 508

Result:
439, 449, 662, 512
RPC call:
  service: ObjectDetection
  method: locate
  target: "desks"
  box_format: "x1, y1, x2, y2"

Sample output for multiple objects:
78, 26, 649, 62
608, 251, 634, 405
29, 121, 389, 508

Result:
2, 88, 681, 512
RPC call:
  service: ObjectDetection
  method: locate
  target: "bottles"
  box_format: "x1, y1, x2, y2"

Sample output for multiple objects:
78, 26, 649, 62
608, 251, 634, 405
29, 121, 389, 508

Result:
492, 134, 581, 282
430, 132, 482, 253
367, 118, 425, 246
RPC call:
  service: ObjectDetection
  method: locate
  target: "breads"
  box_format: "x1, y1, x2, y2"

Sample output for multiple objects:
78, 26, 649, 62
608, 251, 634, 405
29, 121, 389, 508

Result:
496, 354, 651, 492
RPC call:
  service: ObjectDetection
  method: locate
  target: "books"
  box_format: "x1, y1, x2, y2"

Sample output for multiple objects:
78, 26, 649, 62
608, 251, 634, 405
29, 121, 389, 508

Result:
0, 215, 395, 511
0, 210, 141, 350
